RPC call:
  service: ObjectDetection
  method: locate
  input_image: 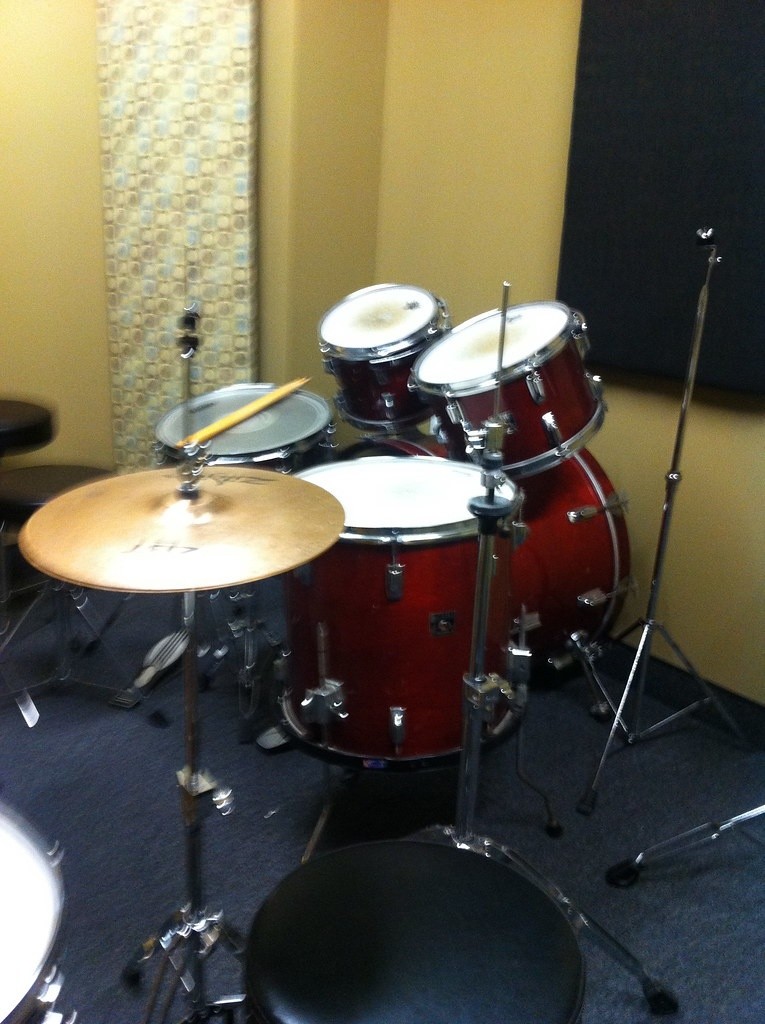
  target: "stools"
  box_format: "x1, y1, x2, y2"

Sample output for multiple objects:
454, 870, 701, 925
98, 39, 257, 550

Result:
0, 397, 129, 708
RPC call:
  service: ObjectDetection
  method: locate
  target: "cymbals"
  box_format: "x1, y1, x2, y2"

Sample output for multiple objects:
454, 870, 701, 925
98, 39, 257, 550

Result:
17, 465, 349, 594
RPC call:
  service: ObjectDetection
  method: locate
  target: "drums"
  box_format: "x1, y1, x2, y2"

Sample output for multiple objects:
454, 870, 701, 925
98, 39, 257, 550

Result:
151, 380, 334, 474
267, 452, 531, 774
336, 428, 634, 686
409, 299, 606, 488
314, 279, 446, 436
